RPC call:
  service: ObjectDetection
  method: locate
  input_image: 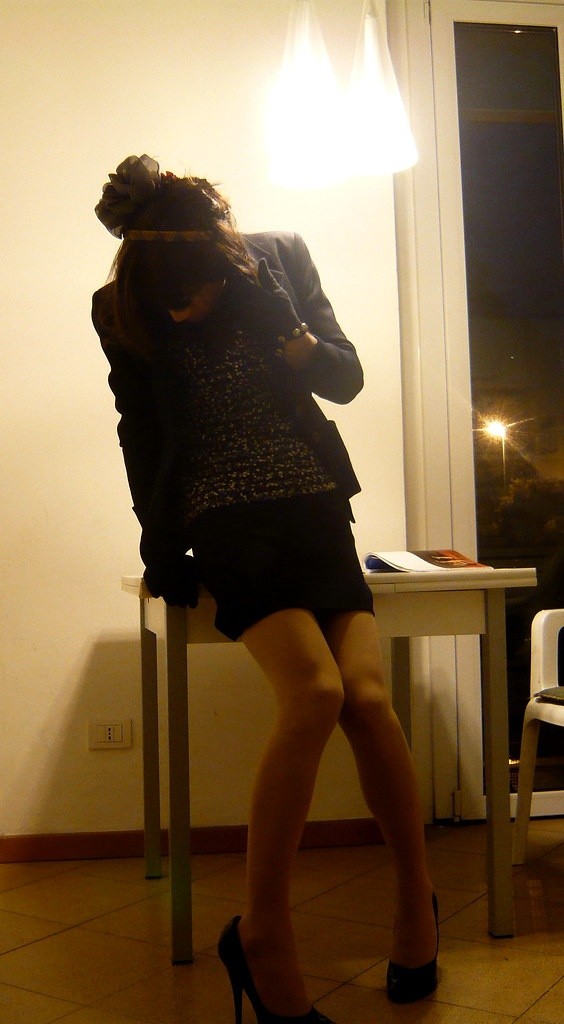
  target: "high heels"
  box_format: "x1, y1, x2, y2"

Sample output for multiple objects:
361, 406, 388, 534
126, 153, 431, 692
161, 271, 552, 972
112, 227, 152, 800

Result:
216, 914, 337, 1024
385, 890, 439, 1003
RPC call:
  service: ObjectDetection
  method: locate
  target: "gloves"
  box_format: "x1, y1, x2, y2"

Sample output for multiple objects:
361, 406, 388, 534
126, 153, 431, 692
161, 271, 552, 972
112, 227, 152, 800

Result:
140, 524, 206, 611
224, 256, 310, 362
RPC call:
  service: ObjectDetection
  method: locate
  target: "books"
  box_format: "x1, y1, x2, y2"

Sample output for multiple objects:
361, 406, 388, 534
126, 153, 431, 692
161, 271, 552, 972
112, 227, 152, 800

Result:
359, 550, 495, 572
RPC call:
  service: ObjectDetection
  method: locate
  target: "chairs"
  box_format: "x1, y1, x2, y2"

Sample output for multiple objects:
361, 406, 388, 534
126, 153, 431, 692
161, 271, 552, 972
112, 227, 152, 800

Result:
510, 608, 564, 865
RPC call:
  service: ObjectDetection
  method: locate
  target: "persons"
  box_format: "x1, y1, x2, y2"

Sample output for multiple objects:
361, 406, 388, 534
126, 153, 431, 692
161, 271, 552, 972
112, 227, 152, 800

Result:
90, 174, 441, 1023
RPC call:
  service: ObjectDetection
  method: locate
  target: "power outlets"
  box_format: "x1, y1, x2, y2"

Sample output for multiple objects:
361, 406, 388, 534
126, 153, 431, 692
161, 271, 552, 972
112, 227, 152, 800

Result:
89, 718, 132, 749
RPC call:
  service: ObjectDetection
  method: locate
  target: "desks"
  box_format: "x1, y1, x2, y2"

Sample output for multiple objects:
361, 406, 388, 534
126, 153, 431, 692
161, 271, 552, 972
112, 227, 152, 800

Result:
121, 567, 538, 965
